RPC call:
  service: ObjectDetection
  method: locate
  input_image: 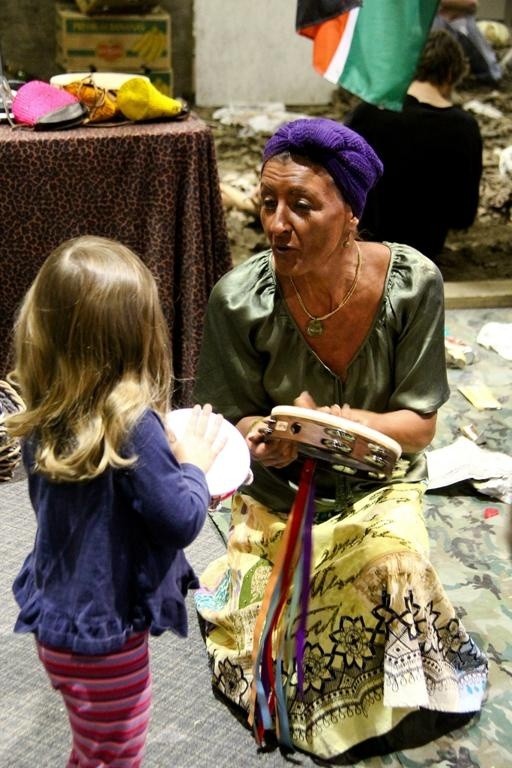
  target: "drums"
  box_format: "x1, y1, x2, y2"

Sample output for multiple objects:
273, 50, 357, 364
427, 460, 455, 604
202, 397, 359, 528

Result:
261, 403, 402, 483
162, 406, 253, 511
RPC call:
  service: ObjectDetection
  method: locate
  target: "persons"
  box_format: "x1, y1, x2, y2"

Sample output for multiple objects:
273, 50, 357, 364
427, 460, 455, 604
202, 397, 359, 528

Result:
6, 235, 227, 768
193, 119, 488, 760
344, 28, 483, 258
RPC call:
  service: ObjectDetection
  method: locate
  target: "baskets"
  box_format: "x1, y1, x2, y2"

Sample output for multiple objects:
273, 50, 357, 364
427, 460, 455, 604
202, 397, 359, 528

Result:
1, 379, 26, 483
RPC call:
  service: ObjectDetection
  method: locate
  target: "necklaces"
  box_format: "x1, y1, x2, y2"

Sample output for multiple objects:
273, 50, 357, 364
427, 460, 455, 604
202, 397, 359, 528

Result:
289, 240, 362, 337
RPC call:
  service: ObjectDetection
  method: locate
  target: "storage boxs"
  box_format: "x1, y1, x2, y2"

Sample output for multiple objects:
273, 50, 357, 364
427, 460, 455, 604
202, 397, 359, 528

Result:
55, 8, 174, 103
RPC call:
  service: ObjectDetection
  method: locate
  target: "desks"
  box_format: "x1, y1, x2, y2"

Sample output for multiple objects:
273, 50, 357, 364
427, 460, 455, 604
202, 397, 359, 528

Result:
1, 79, 212, 404
